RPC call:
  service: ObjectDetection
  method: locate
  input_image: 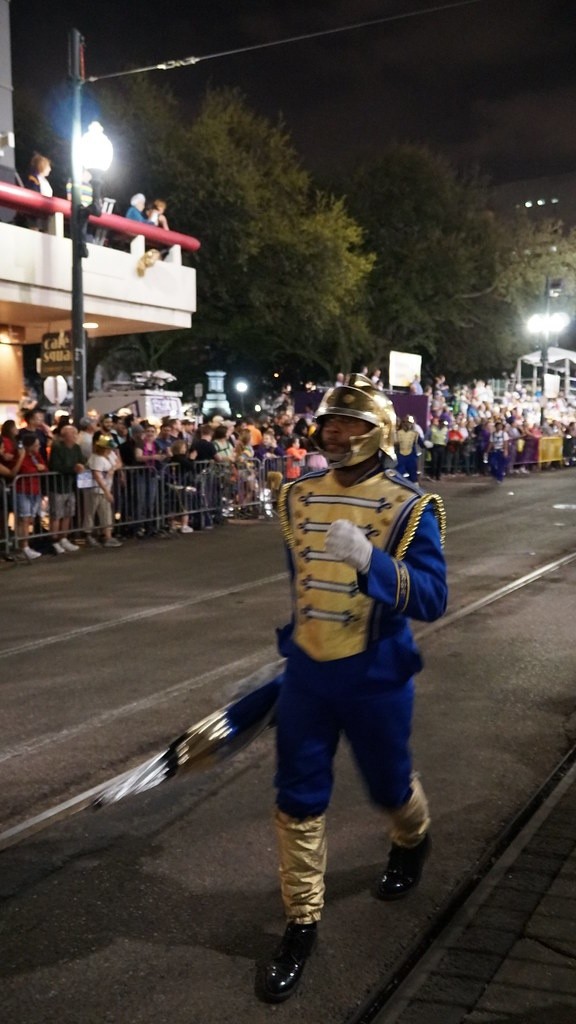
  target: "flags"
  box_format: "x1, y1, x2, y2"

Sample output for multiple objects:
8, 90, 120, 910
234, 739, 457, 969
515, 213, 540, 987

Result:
94, 670, 291, 815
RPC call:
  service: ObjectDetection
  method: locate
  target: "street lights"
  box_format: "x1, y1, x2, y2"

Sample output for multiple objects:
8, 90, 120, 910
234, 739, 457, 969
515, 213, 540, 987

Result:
235, 380, 248, 413
539, 276, 562, 432
72, 28, 114, 427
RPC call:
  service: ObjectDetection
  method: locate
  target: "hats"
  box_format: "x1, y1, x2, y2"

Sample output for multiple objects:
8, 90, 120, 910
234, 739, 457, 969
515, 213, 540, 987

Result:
80, 416, 95, 426
96, 436, 118, 449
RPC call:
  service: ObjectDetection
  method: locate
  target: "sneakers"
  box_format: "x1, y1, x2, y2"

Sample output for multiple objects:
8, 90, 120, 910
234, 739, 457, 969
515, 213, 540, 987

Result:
61, 539, 80, 551
53, 543, 64, 554
181, 525, 194, 533
18, 547, 42, 560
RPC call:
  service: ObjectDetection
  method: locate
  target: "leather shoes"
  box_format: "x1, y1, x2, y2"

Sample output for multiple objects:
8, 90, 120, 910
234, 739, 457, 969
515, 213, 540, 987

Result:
378, 832, 432, 901
261, 920, 318, 1000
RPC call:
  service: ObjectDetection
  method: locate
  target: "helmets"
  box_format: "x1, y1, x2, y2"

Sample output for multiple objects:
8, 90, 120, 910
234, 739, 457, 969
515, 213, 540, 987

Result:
402, 415, 414, 424
307, 373, 397, 467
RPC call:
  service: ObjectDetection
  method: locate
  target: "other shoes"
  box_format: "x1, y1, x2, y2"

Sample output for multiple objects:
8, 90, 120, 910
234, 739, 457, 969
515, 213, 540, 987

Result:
512, 468, 529, 475
104, 538, 122, 547
85, 539, 102, 550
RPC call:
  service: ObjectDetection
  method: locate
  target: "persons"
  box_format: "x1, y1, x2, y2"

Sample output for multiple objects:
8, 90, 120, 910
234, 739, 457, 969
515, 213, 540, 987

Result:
0, 379, 576, 565
256, 370, 450, 1004
6, 150, 54, 231
126, 190, 174, 259
59, 169, 102, 244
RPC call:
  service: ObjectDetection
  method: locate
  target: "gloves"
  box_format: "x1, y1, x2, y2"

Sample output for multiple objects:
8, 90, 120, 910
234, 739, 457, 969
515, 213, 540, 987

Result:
324, 520, 373, 572
